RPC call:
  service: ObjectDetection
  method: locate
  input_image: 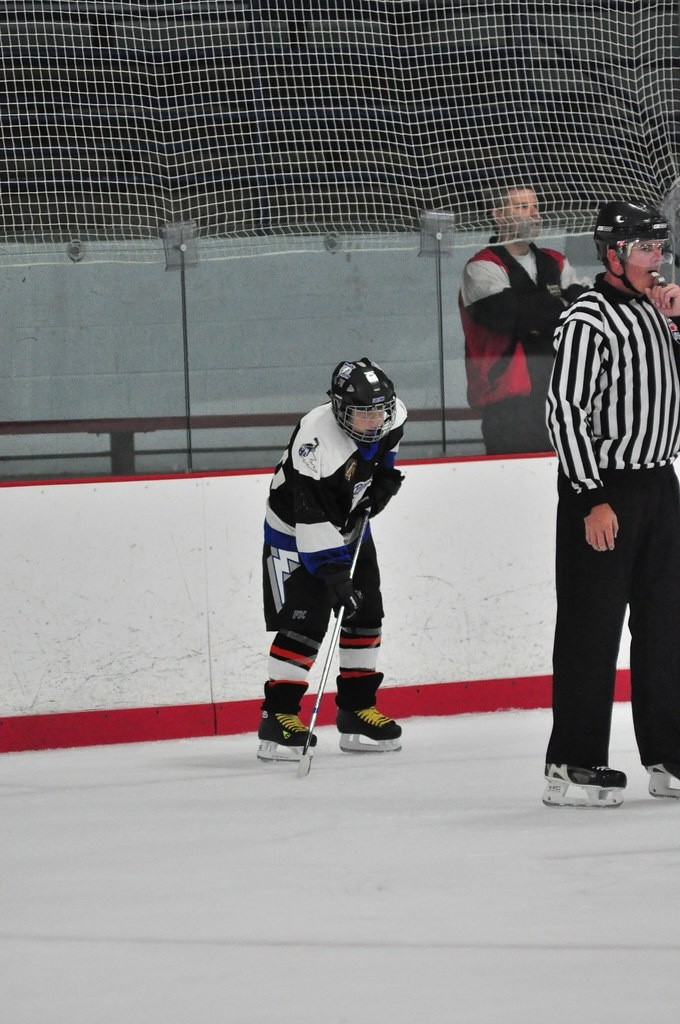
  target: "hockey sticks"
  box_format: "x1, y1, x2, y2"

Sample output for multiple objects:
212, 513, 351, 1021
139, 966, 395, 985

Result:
290, 498, 375, 782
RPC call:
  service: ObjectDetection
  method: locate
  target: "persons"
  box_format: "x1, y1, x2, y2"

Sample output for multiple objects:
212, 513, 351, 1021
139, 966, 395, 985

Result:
258, 357, 409, 760
543, 205, 680, 810
458, 185, 594, 455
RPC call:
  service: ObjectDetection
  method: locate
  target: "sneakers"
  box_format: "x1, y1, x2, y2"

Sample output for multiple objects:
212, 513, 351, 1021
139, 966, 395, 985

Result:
647, 764, 680, 802
541, 760, 629, 808
257, 710, 317, 763
335, 707, 403, 753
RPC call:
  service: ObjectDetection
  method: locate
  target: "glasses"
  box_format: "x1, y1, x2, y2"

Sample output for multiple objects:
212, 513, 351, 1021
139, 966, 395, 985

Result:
628, 242, 667, 255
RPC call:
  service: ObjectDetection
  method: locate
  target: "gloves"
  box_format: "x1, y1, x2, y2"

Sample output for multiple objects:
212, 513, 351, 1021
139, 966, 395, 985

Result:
356, 466, 405, 517
315, 562, 362, 620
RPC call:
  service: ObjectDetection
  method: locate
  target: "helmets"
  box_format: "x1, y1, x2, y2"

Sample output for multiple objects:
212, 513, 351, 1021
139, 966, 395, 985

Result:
327, 356, 397, 443
592, 200, 671, 261
